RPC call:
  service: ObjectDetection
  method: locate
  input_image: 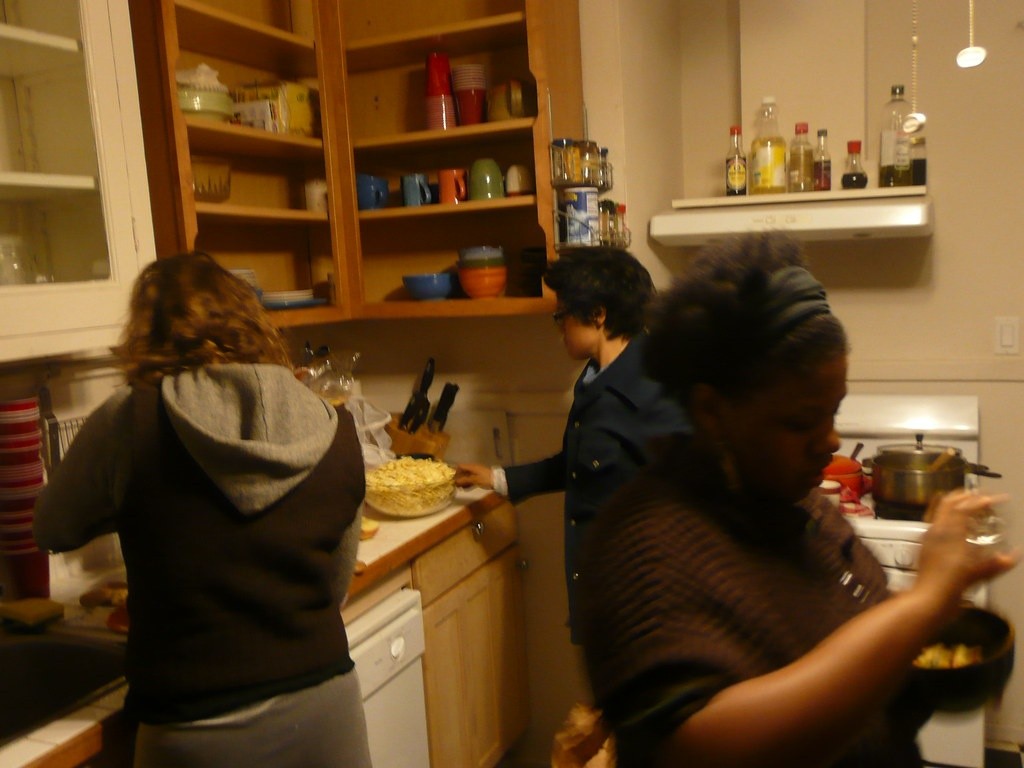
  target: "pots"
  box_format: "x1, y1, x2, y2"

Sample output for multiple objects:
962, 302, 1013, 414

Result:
861, 433, 999, 514
823, 443, 864, 502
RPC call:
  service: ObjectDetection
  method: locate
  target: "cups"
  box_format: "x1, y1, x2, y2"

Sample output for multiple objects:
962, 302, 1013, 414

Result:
355, 157, 533, 209
423, 49, 489, 131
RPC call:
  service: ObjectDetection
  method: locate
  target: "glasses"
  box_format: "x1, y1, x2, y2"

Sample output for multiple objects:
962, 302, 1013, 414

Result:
551, 305, 585, 325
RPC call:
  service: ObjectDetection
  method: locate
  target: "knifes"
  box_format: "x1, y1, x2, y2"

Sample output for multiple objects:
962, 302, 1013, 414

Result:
395, 356, 458, 437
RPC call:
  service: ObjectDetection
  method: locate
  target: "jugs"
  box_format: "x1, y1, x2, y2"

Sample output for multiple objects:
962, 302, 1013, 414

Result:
489, 79, 535, 121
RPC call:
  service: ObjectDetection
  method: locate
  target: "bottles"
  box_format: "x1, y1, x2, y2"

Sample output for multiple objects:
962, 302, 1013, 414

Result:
548, 138, 631, 251
724, 82, 926, 197
0, 234, 30, 287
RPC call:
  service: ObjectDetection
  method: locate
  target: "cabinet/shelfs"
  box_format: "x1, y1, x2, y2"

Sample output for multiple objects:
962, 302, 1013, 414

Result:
340, 567, 430, 768
322, 0, 586, 326
409, 504, 529, 767
0, 1, 157, 361
130, 2, 351, 327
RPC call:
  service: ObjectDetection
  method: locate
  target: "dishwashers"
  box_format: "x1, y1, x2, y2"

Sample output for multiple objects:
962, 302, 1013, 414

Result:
346, 587, 430, 768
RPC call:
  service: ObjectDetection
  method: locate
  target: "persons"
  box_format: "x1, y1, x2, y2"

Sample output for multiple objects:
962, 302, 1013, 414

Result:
572, 237, 1024, 768
34, 253, 371, 768
448, 246, 656, 730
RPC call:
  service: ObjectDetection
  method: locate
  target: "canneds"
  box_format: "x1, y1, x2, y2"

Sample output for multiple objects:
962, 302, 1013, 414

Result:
551, 138, 625, 245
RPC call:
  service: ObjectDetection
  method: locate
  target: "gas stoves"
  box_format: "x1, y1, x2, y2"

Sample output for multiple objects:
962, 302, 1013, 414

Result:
818, 393, 980, 572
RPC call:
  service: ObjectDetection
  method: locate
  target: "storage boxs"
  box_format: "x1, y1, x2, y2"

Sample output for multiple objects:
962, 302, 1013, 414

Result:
232, 79, 321, 137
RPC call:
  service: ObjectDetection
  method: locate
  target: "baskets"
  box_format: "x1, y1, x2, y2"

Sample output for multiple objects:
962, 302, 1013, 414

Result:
363, 465, 460, 519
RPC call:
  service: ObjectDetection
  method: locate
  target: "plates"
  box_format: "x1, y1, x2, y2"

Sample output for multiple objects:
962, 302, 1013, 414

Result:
229, 268, 314, 306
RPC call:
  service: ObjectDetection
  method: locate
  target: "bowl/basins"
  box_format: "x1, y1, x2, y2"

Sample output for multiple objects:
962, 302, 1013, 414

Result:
365, 466, 459, 516
401, 245, 545, 301
899, 607, 1016, 714
